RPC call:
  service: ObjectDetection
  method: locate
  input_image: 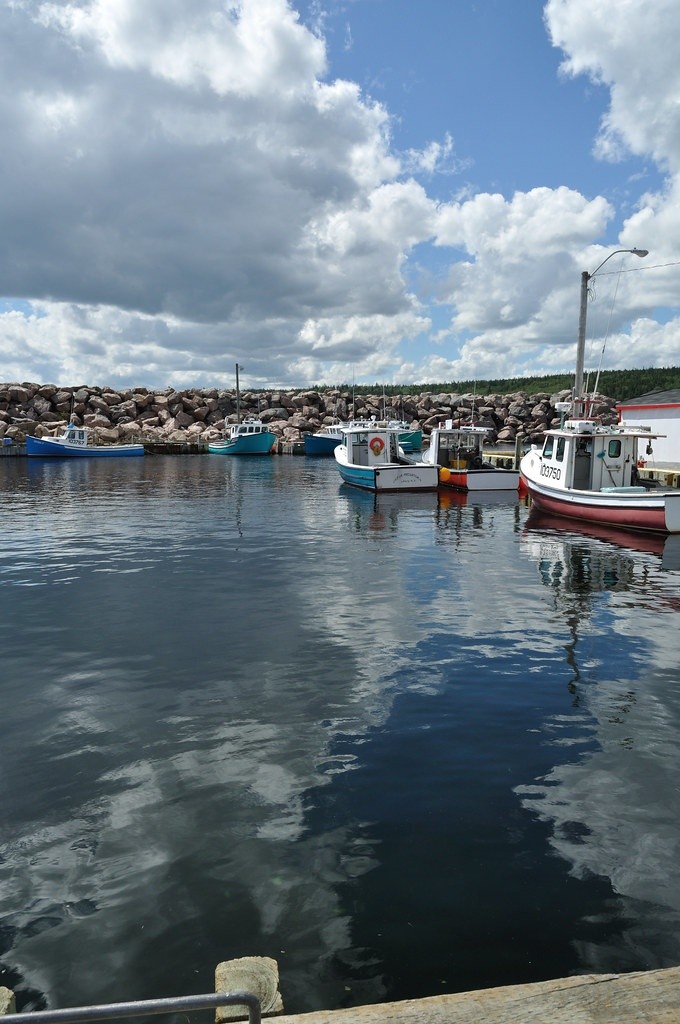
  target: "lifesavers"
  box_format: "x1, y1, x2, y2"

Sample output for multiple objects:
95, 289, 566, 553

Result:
370, 438, 384, 451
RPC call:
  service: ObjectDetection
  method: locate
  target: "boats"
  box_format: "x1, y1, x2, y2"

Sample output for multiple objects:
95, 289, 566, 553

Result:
206, 361, 276, 454
303, 419, 423, 456
518, 397, 680, 538
421, 418, 522, 493
24, 422, 147, 459
332, 418, 442, 492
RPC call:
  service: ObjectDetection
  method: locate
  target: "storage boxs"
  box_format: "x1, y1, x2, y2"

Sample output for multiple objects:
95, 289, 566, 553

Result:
449, 460, 467, 468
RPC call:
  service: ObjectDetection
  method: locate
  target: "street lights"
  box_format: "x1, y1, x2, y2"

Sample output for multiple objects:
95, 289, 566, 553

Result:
571, 248, 651, 420
